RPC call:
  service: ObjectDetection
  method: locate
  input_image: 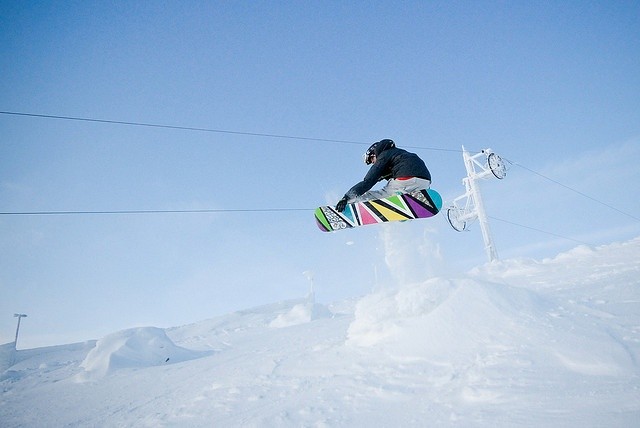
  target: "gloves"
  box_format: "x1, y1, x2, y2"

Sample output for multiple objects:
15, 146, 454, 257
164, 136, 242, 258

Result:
335, 194, 350, 212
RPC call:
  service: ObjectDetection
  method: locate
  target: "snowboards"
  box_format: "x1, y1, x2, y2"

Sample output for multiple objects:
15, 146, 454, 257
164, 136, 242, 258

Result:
313, 188, 443, 233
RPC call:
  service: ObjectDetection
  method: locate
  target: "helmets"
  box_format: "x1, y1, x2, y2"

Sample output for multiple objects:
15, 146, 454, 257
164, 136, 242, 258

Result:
364, 142, 379, 165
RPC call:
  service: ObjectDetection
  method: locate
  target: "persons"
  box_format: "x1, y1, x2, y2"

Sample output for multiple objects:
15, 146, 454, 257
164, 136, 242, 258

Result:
335, 139, 431, 212
